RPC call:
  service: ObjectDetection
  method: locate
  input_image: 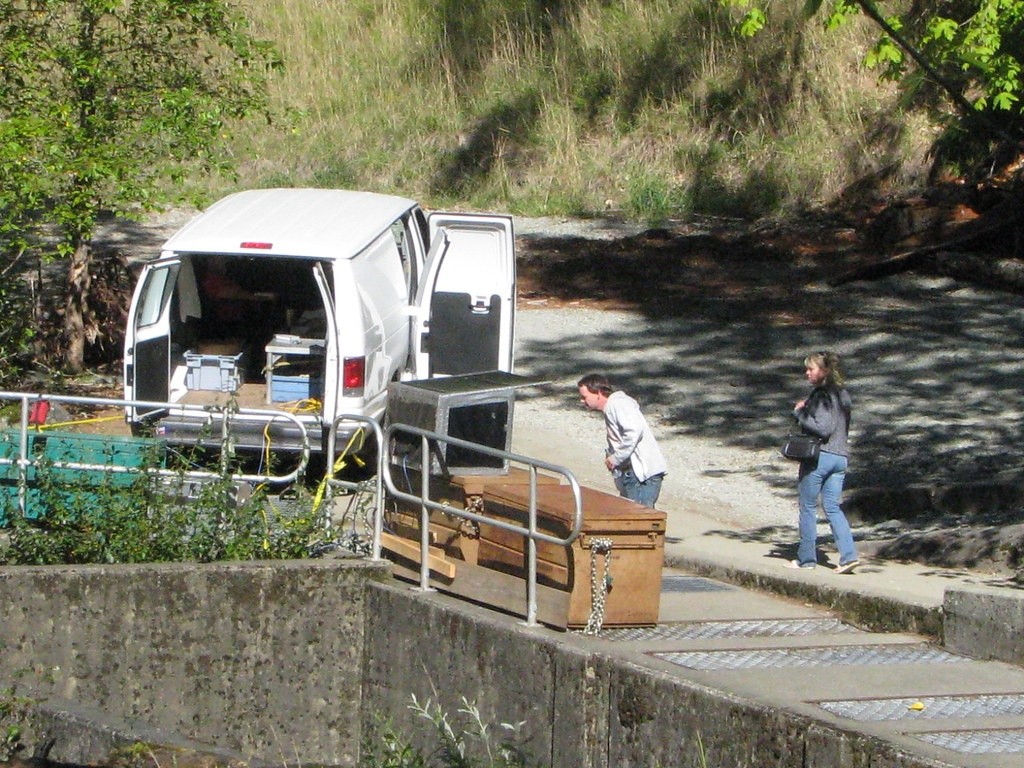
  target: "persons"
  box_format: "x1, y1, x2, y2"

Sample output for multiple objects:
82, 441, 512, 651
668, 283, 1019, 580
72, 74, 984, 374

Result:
578, 373, 667, 509
782, 352, 860, 574
193, 253, 273, 380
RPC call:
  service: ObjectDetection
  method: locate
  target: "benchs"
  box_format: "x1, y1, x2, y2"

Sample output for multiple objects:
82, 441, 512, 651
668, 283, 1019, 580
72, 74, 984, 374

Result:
0, 427, 167, 526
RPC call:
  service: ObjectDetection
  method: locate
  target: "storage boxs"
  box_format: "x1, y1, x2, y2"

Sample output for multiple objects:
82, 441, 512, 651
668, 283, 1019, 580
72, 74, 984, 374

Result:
183, 346, 247, 393
271, 373, 320, 403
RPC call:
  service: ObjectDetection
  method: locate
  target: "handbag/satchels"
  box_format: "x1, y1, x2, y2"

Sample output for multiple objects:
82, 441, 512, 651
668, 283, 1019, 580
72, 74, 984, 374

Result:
780, 432, 823, 463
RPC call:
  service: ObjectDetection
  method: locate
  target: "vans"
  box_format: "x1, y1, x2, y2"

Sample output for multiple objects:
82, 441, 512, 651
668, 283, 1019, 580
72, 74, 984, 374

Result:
109, 185, 515, 485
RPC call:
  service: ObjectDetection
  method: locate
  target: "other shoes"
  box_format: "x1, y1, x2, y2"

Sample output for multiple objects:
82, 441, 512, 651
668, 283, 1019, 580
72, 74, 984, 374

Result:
783, 557, 816, 570
832, 561, 860, 573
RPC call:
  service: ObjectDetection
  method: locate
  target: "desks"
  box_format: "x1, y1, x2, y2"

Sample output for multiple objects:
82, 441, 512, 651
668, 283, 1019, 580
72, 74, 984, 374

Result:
265, 336, 326, 406
